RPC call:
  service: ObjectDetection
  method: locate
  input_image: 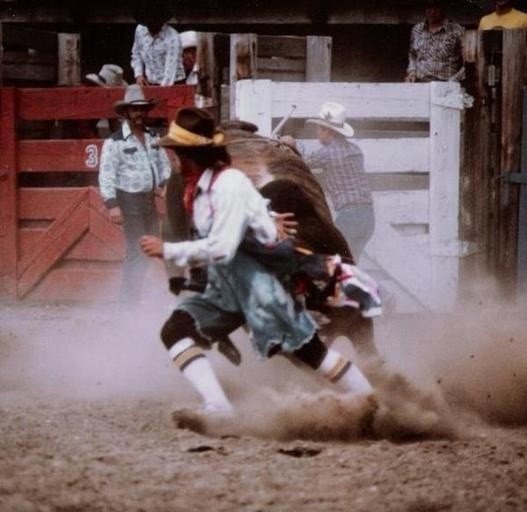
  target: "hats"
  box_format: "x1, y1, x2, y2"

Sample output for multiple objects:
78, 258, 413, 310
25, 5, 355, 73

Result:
85, 64, 129, 86
306, 102, 354, 137
115, 85, 157, 116
152, 107, 227, 155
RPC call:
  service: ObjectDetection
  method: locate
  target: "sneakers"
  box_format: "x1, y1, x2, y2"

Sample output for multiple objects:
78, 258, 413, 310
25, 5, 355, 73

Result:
172, 407, 241, 440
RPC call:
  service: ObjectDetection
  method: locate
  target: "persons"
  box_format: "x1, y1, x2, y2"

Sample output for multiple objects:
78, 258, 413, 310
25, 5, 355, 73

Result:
98, 83, 174, 315
60, 64, 130, 187
130, 7, 188, 87
473, 1, 526, 31
403, 1, 470, 83
182, 42, 201, 87
132, 106, 387, 421
278, 100, 374, 271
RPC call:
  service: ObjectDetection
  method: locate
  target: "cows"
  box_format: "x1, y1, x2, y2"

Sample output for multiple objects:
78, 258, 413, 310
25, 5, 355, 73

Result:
164, 117, 377, 366
